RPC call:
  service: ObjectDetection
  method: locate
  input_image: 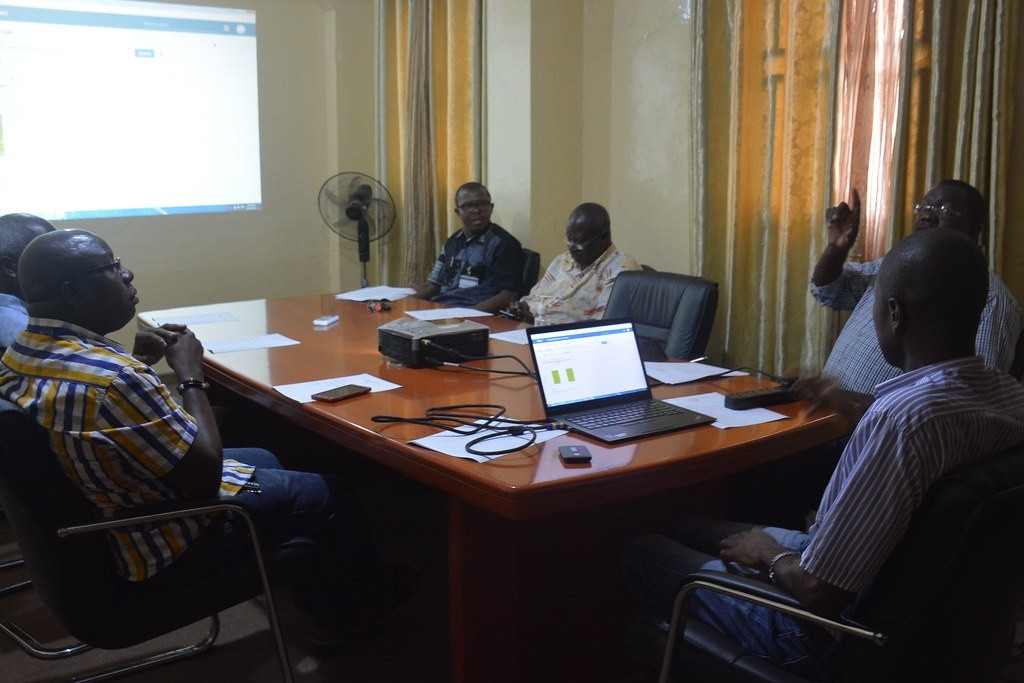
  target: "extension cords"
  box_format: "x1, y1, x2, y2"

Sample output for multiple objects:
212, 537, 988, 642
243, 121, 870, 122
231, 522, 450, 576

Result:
725, 383, 798, 412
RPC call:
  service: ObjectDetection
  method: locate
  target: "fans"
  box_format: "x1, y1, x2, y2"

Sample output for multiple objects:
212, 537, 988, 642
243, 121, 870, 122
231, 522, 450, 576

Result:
317, 172, 396, 288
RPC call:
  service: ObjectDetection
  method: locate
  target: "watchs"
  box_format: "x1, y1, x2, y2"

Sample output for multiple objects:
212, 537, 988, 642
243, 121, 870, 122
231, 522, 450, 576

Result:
768, 551, 801, 590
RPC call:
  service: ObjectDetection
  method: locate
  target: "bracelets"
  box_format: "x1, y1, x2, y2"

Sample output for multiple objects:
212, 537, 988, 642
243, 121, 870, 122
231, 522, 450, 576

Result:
177, 379, 211, 395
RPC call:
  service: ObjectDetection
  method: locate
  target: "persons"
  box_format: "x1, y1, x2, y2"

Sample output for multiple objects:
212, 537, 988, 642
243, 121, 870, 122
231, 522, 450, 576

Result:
1, 214, 243, 449
409, 182, 523, 314
0, 230, 422, 648
506, 204, 644, 326
810, 179, 1024, 399
621, 227, 1024, 675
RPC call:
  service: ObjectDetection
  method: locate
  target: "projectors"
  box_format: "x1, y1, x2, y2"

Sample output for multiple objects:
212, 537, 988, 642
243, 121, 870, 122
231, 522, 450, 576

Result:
377, 317, 491, 366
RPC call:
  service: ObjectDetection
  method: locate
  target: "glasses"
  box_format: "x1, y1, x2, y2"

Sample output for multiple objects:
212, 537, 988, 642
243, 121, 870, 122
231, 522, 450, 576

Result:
57, 256, 121, 291
563, 229, 608, 251
913, 203, 976, 223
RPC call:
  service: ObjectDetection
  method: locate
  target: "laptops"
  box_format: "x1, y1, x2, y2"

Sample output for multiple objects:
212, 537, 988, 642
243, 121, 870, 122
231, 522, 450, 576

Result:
526, 317, 718, 446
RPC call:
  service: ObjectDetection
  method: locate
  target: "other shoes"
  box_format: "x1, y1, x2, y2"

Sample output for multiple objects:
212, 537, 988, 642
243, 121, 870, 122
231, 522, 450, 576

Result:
310, 607, 387, 649
620, 618, 668, 668
344, 563, 419, 606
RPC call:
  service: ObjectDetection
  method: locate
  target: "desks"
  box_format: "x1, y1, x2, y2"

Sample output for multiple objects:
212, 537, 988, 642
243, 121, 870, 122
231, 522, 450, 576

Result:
138, 287, 876, 683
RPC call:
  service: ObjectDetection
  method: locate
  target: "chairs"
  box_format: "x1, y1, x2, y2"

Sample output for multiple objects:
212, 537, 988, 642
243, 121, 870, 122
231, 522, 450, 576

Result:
0, 395, 295, 682
603, 270, 720, 361
622, 440, 1024, 683
520, 247, 541, 300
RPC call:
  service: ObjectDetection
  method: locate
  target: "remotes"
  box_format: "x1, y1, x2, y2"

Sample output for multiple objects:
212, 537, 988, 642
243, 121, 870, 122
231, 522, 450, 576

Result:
313, 314, 340, 326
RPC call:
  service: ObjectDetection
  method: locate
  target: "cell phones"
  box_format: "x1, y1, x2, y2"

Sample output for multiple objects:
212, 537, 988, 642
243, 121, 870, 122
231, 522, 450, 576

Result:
501, 309, 523, 322
559, 444, 592, 464
310, 384, 372, 403
